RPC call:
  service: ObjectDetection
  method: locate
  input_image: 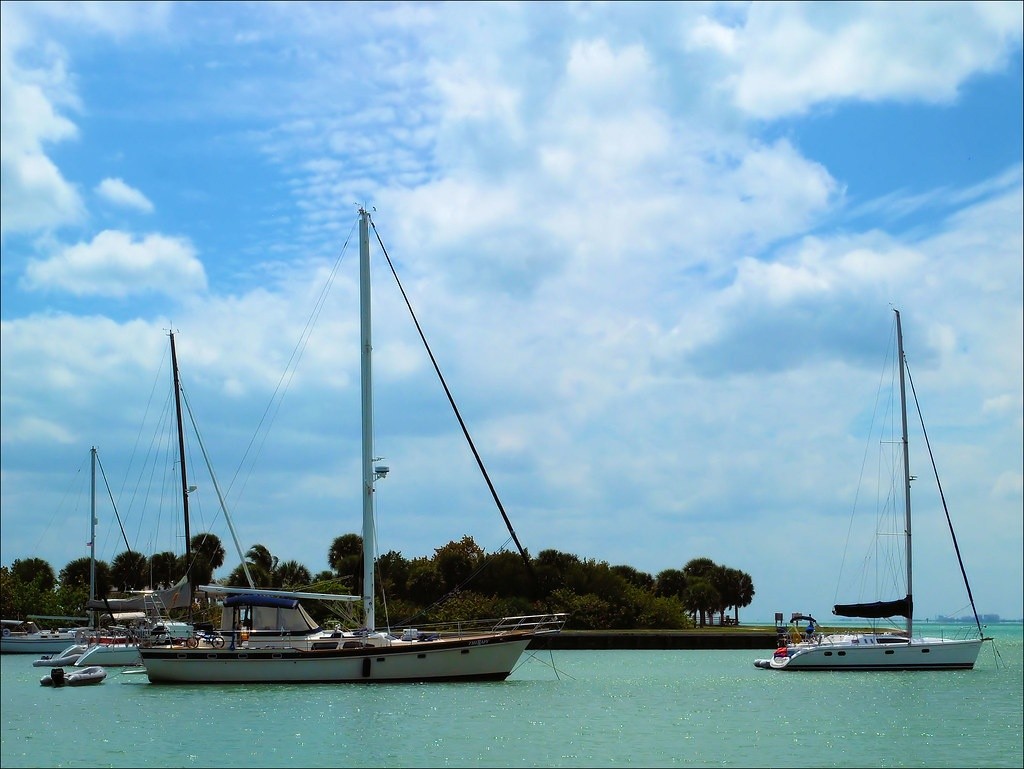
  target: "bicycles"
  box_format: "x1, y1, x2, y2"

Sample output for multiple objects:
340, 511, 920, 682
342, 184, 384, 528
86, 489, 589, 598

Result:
186, 630, 225, 649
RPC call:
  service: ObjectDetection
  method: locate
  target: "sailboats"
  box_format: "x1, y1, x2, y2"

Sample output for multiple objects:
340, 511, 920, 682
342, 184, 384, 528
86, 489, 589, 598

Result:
139, 200, 570, 686
754, 296, 993, 671
1, 325, 257, 667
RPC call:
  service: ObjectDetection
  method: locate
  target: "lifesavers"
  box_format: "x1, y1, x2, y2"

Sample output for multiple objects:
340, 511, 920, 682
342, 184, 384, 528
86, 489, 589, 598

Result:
3, 628, 10, 637
793, 633, 801, 643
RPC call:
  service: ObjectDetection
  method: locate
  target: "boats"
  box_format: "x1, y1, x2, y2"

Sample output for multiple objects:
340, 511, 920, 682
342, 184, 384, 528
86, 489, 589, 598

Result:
41, 666, 107, 686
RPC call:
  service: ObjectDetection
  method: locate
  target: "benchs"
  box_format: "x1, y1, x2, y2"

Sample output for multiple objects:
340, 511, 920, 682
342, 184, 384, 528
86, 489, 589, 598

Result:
723, 618, 739, 626
